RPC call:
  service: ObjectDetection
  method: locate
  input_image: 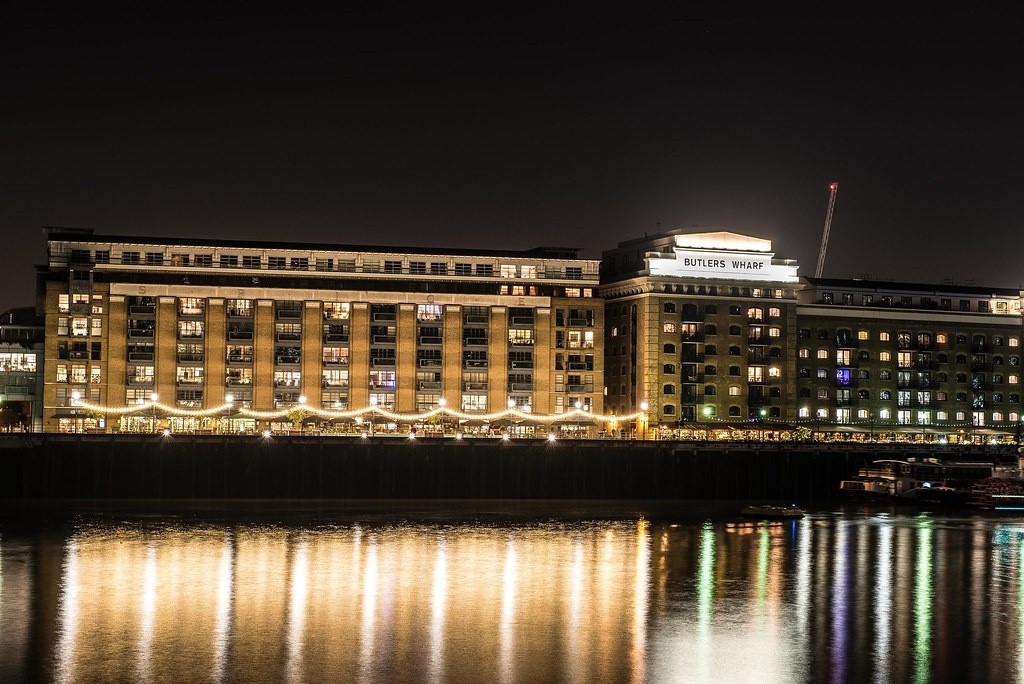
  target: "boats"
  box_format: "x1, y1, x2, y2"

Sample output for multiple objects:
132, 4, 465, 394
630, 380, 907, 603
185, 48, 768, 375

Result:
892, 459, 1024, 522
840, 457, 993, 506
741, 502, 804, 523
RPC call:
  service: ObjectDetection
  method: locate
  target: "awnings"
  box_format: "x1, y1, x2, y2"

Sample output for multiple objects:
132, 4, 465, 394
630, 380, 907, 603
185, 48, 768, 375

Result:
659, 422, 1015, 434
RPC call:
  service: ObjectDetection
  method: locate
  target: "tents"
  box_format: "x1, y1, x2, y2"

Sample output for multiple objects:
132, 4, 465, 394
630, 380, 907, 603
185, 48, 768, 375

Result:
122, 404, 598, 438
